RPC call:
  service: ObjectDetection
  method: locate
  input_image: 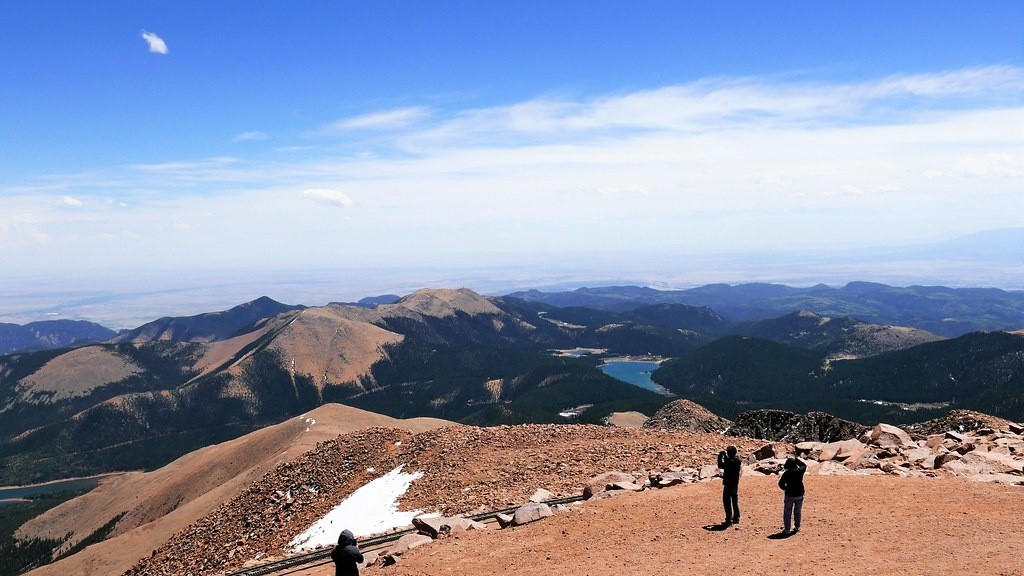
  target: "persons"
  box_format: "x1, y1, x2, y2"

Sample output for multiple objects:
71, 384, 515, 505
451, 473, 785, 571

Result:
717, 445, 742, 525
777, 455, 807, 536
331, 529, 364, 576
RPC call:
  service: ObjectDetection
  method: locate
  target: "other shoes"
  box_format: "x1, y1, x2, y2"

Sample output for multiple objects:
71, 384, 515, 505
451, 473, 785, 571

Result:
783, 528, 790, 533
795, 525, 800, 530
731, 518, 739, 524
722, 522, 732, 526
